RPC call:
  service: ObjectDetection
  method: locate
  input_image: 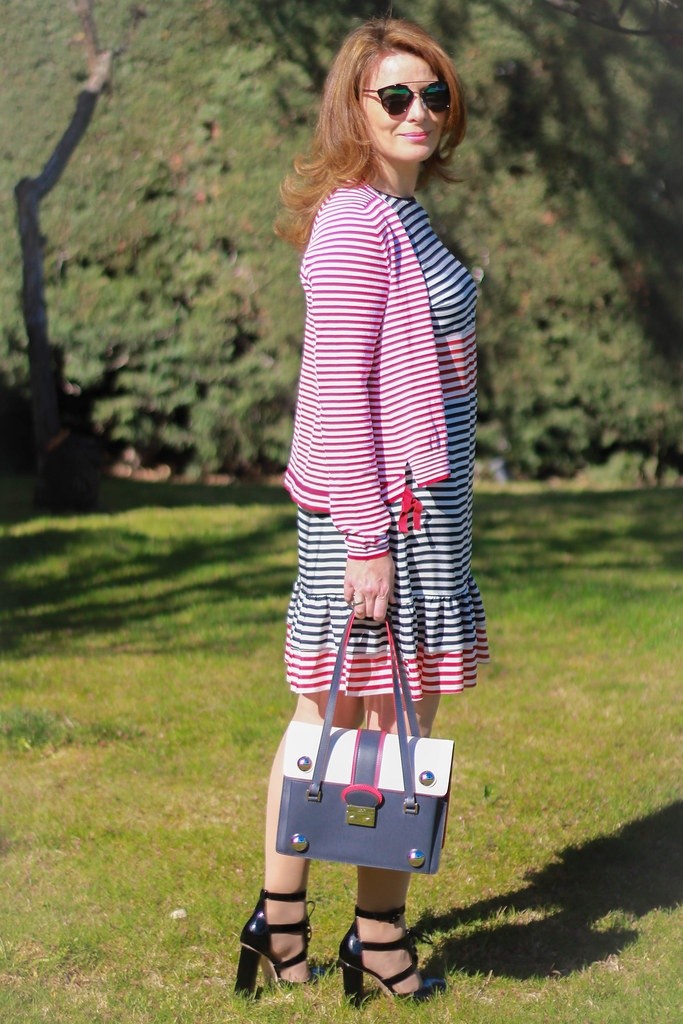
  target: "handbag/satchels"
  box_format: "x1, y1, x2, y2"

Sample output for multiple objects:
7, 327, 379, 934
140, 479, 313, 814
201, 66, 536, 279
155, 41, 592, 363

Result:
275, 608, 456, 875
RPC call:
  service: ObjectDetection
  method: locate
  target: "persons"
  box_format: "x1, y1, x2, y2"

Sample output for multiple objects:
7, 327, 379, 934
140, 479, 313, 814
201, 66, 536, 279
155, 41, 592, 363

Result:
233, 19, 491, 1005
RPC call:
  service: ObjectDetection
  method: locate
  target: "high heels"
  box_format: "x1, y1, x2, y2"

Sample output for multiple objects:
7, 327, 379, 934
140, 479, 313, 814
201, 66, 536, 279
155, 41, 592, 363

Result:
233, 887, 323, 999
336, 903, 447, 1012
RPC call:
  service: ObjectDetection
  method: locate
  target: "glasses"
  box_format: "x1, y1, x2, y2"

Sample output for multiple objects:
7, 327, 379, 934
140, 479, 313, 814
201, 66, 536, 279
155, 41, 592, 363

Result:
359, 80, 452, 116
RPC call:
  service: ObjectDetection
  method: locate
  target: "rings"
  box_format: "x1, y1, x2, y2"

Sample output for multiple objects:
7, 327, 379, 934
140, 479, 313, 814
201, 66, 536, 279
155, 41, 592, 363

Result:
352, 602, 363, 606
